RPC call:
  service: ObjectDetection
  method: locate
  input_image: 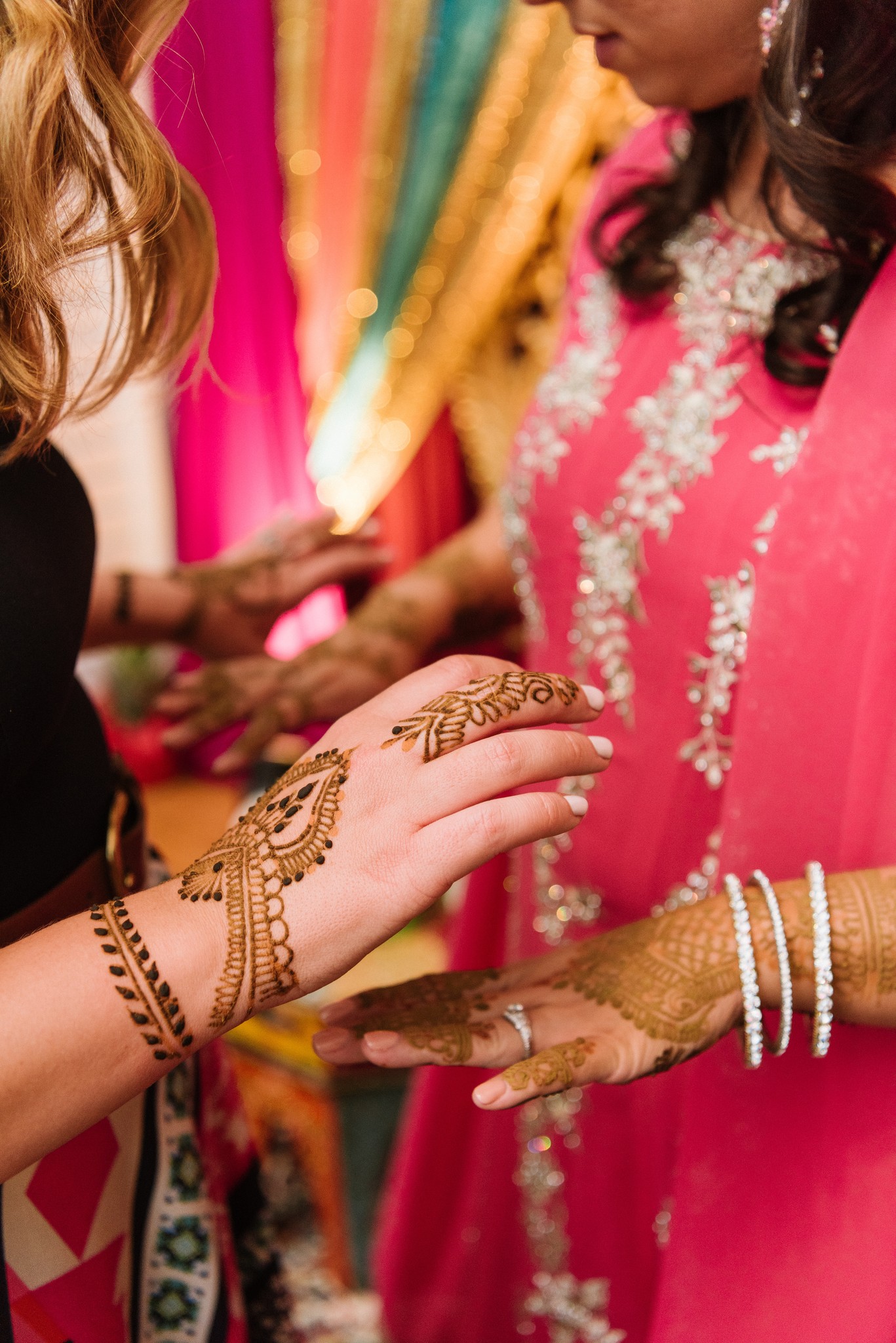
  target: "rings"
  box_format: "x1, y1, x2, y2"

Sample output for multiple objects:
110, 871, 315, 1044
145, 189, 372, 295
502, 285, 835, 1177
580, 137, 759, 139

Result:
503, 1004, 533, 1060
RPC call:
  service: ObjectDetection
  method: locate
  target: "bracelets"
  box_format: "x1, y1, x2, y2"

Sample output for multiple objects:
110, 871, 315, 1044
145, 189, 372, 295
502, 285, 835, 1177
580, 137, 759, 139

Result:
723, 861, 833, 1069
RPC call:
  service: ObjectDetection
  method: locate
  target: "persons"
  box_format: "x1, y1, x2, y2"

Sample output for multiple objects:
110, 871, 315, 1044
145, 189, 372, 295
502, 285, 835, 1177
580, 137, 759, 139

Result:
0, 0, 611, 1343
157, 0, 896, 1343
